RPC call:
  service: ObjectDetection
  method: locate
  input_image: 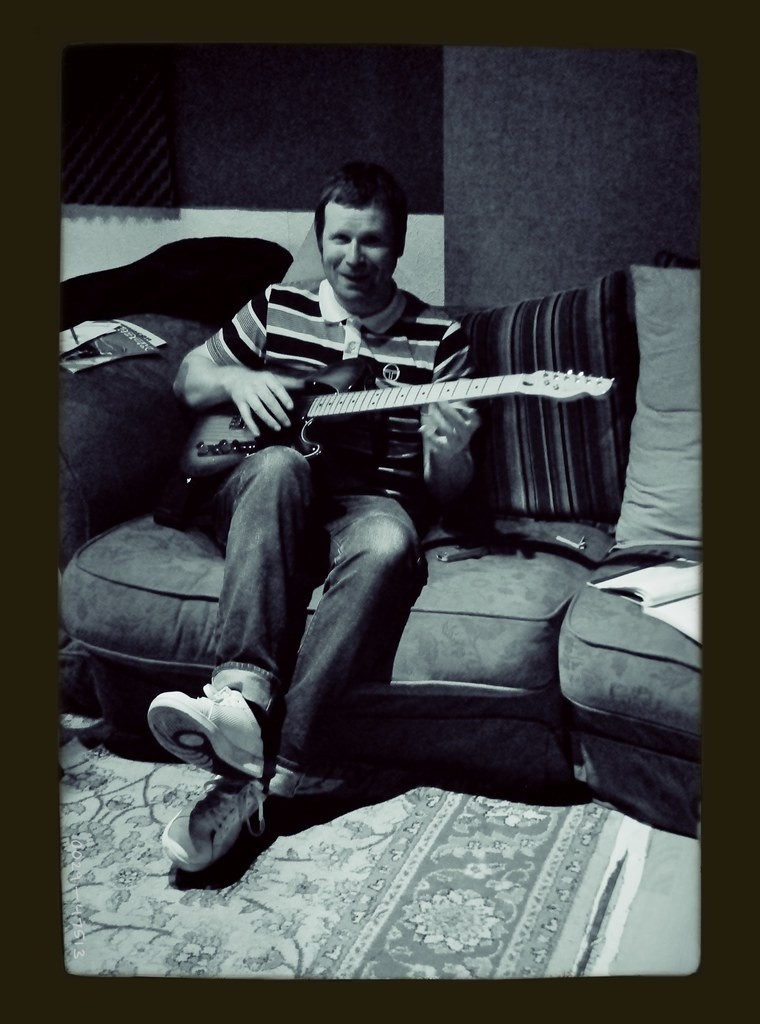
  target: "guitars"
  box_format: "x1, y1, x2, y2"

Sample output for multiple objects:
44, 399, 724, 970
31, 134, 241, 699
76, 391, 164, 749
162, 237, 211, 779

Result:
180, 359, 615, 477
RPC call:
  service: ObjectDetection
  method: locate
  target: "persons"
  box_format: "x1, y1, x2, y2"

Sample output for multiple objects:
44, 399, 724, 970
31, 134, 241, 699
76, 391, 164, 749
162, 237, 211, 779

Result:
147, 162, 482, 873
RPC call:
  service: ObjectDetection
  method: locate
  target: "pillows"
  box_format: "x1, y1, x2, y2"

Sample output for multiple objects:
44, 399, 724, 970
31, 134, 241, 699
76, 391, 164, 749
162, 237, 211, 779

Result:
617, 263, 702, 549
279, 211, 446, 307
426, 270, 637, 527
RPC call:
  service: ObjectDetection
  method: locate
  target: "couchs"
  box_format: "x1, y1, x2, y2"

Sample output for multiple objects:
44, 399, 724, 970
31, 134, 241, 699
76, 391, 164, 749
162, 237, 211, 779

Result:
558, 551, 701, 835
60, 312, 614, 776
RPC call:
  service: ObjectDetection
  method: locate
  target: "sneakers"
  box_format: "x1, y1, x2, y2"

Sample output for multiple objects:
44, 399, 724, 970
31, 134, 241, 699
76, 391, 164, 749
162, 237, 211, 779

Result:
147, 683, 263, 780
161, 773, 266, 871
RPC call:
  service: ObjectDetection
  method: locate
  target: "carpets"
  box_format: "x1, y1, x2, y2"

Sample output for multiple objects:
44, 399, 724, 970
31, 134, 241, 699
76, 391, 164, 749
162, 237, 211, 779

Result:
61, 715, 651, 977
